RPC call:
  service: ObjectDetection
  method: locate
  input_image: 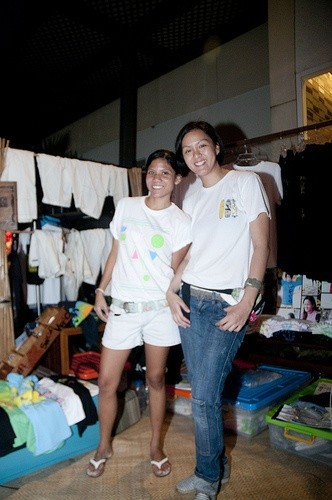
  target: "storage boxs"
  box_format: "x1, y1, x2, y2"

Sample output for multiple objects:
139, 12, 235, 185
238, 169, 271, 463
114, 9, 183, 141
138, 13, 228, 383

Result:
73, 352, 130, 392
4, 306, 71, 375
167, 359, 332, 464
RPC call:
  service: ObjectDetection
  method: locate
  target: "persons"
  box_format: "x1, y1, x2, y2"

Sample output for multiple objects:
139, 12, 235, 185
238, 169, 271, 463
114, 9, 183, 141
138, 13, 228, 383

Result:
302, 296, 320, 323
167, 121, 272, 500
87, 149, 192, 477
277, 271, 303, 307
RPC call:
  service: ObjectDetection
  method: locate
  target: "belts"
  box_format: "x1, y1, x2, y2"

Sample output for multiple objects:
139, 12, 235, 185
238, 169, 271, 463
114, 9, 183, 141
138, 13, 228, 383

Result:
111, 299, 169, 314
179, 283, 224, 302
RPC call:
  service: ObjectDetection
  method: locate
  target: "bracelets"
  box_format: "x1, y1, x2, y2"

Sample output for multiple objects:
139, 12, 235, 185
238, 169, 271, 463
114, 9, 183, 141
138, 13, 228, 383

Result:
244, 278, 262, 289
95, 288, 105, 295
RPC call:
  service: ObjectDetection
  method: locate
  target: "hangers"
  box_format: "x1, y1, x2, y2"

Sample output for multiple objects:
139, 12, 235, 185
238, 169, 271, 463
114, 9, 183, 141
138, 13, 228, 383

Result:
235, 139, 260, 165
274, 128, 328, 155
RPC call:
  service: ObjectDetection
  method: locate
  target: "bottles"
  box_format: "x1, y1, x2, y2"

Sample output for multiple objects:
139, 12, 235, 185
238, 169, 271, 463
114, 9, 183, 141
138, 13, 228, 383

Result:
134, 381, 146, 410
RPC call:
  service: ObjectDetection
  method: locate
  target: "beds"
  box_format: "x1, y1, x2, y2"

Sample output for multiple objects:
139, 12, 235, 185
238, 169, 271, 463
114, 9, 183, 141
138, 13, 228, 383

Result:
0, 380, 100, 484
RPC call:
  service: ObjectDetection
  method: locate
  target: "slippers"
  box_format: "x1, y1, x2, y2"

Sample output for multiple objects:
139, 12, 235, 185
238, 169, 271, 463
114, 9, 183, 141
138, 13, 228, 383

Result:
87, 457, 106, 477
150, 456, 171, 477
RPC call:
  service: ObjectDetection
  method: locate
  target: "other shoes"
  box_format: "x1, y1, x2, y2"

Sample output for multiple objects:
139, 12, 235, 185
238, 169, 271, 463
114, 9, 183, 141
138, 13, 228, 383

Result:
194, 491, 211, 500
176, 473, 230, 494
